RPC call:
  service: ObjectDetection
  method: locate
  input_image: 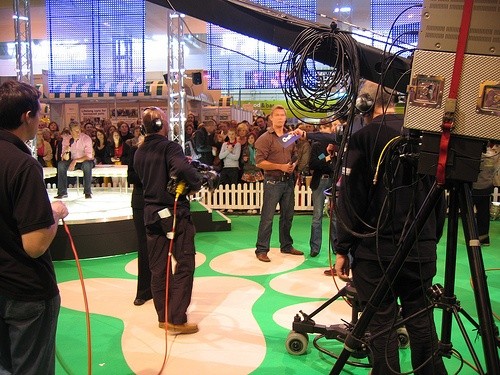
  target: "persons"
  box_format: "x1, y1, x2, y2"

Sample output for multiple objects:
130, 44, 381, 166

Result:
172, 112, 271, 215
0, 82, 70, 375
25, 119, 74, 189
133, 106, 203, 333
278, 76, 500, 375
121, 134, 153, 306
77, 118, 145, 188
53, 121, 96, 199
253, 106, 303, 262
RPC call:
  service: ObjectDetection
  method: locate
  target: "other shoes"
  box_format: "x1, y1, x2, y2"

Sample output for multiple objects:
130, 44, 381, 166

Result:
133, 296, 145, 305
280, 246, 304, 255
479, 236, 490, 246
256, 250, 271, 263
247, 209, 258, 214
227, 209, 233, 213
310, 249, 318, 257
159, 321, 199, 335
85, 193, 92, 199
54, 194, 68, 199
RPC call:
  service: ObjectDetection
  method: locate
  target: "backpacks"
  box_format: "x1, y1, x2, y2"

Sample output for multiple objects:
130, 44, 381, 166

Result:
185, 130, 203, 160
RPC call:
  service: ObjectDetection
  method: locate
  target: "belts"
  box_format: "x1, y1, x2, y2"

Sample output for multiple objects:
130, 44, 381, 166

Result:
320, 174, 330, 179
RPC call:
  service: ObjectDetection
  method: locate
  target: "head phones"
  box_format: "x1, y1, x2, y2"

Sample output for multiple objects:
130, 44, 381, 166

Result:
359, 78, 398, 112
139, 106, 162, 136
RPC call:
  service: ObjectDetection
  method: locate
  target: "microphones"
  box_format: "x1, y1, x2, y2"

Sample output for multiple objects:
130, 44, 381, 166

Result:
289, 154, 298, 164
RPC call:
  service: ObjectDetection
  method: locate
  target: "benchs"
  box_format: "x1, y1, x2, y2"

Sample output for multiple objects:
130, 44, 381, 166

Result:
43, 165, 128, 192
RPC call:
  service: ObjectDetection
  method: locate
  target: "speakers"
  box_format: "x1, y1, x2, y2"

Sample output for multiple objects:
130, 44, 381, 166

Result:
192, 72, 201, 85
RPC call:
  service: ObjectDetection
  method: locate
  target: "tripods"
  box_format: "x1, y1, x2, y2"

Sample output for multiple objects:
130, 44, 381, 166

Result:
325, 131, 500, 375
